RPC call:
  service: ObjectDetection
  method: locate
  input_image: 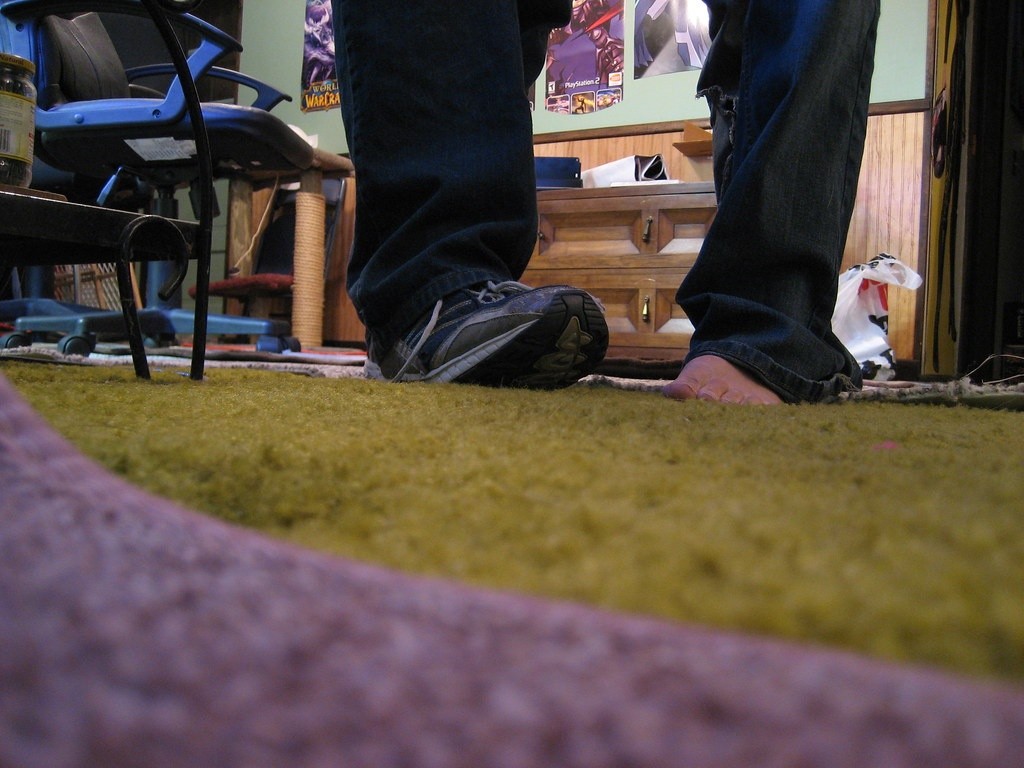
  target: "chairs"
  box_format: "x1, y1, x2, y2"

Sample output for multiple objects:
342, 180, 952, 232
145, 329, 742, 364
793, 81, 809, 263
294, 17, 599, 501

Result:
0, 0, 315, 381
0, 0, 214, 381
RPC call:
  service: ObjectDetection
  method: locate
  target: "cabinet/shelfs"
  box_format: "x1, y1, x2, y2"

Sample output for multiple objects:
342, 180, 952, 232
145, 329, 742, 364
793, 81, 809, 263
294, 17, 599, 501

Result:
517, 181, 718, 360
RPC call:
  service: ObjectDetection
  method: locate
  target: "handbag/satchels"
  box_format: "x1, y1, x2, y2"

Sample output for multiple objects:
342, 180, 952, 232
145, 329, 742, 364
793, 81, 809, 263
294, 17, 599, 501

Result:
830, 252, 923, 381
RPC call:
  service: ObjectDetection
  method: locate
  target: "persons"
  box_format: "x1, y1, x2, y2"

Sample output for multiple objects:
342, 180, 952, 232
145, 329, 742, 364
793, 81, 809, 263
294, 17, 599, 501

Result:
330, 0, 882, 409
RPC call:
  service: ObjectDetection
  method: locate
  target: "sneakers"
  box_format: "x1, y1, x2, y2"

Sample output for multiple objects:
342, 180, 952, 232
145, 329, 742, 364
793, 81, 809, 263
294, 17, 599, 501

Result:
362, 280, 609, 392
